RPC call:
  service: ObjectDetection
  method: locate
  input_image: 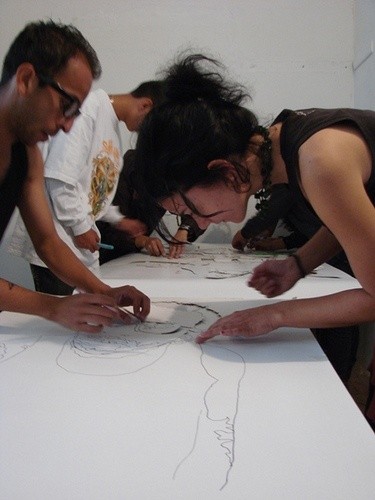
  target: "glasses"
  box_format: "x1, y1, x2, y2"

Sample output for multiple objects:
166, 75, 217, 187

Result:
40, 75, 82, 119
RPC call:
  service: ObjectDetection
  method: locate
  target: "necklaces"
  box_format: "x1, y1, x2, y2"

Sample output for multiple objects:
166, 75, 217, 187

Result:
250, 126, 274, 225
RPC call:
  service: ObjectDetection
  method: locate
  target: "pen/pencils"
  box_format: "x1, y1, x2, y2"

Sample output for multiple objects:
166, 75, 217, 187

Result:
105, 303, 142, 324
165, 253, 171, 257
97, 243, 114, 251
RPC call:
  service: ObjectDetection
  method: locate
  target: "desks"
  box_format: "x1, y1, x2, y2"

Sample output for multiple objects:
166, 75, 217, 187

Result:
73, 242, 362, 300
0, 299, 375, 500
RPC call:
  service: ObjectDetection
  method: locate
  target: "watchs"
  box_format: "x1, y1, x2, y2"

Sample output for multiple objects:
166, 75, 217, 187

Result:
177, 223, 192, 231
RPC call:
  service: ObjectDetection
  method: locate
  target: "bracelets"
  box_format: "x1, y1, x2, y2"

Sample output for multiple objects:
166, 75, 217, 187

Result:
288, 253, 306, 278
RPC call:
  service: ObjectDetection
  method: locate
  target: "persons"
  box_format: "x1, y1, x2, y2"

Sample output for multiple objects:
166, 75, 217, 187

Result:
0, 18, 204, 331
134, 51, 375, 345
